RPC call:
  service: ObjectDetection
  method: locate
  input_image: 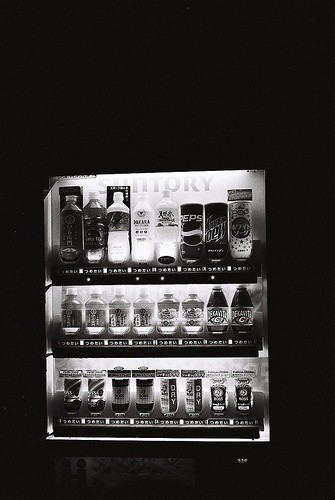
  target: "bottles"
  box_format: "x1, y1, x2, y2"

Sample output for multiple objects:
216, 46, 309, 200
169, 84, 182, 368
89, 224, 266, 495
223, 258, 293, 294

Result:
210, 377, 228, 416
85, 292, 108, 336
230, 285, 253, 339
133, 287, 156, 336
108, 287, 132, 336
83, 191, 107, 266
182, 291, 205, 336
58, 194, 84, 265
131, 191, 154, 263
207, 286, 230, 335
235, 377, 254, 416
154, 189, 180, 265
106, 192, 130, 264
61, 288, 82, 336
157, 289, 180, 336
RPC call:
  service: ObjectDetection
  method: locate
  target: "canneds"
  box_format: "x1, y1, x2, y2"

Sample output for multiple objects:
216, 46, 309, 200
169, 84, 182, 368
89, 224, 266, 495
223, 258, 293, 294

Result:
179, 201, 252, 260
63, 377, 203, 415
